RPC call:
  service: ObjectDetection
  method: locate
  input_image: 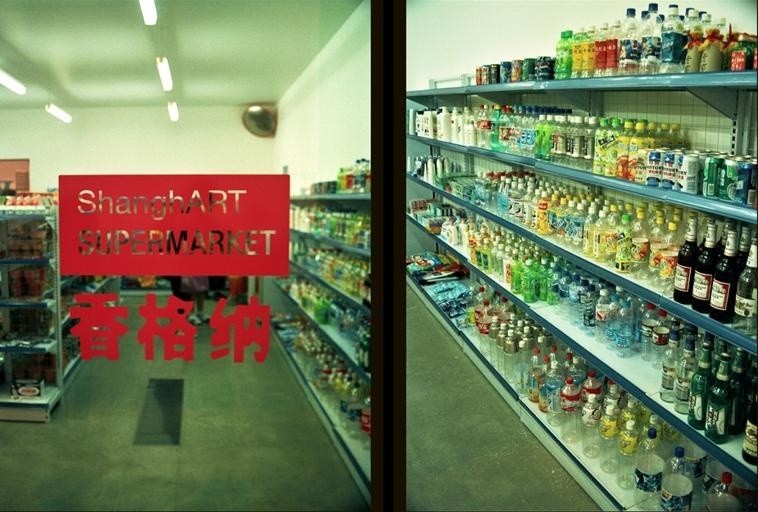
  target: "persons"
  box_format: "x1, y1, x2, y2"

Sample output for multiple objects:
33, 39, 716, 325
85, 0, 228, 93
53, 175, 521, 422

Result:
182, 274, 209, 324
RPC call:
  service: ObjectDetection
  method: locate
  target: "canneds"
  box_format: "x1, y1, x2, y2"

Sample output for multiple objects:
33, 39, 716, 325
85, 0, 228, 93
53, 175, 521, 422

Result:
648, 148, 758, 209
476, 55, 553, 84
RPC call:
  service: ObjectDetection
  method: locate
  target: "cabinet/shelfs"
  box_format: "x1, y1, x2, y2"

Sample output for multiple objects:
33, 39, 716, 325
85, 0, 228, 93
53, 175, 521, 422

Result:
406, 71, 758, 512
0, 203, 125, 425
255, 194, 372, 512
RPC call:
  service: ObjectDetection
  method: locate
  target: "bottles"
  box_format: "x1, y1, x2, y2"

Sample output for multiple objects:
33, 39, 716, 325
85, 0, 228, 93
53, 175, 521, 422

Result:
289, 240, 371, 306
468, 2, 758, 510
286, 279, 369, 370
309, 158, 369, 192
290, 206, 371, 248
296, 328, 371, 450
